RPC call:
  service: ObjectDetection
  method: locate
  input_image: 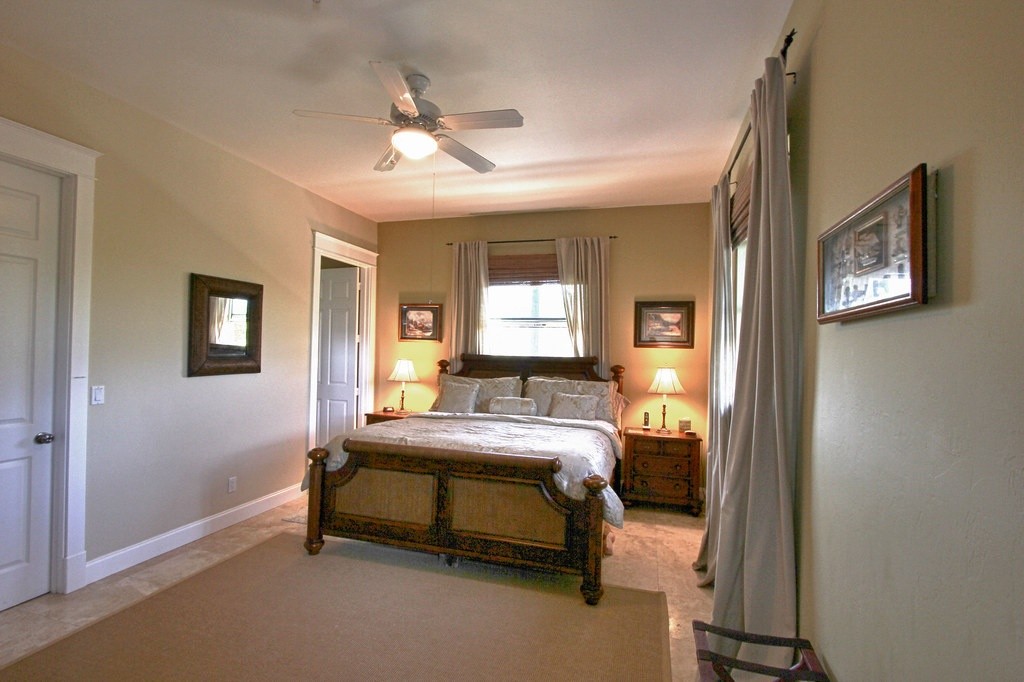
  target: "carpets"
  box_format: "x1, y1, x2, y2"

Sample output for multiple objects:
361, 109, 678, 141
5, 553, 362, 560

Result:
0, 531, 672, 682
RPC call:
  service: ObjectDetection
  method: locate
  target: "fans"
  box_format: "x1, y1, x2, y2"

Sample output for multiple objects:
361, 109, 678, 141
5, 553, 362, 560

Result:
293, 59, 524, 174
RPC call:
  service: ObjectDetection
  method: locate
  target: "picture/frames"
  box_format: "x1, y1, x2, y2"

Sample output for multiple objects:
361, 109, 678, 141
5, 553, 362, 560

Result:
398, 303, 442, 343
816, 163, 928, 325
634, 301, 695, 349
187, 274, 264, 378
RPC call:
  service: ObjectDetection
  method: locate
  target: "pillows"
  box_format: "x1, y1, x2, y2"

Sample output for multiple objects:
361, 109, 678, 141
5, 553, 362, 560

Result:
613, 393, 631, 430
488, 396, 538, 416
519, 380, 523, 396
524, 376, 619, 423
437, 382, 480, 413
428, 373, 520, 413
549, 391, 600, 420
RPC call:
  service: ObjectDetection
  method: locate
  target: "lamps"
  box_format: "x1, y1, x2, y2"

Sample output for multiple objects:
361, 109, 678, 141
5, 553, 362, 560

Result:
647, 367, 686, 434
387, 359, 420, 415
391, 127, 438, 161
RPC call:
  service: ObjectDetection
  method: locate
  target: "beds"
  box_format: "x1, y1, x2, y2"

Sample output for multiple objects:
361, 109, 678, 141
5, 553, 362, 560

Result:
302, 410, 622, 606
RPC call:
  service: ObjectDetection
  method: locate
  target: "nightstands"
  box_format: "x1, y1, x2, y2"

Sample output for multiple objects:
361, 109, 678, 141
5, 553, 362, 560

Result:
365, 410, 415, 426
620, 427, 703, 516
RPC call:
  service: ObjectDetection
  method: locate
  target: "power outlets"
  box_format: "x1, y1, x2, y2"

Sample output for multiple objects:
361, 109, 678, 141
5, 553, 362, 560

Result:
228, 476, 237, 493
